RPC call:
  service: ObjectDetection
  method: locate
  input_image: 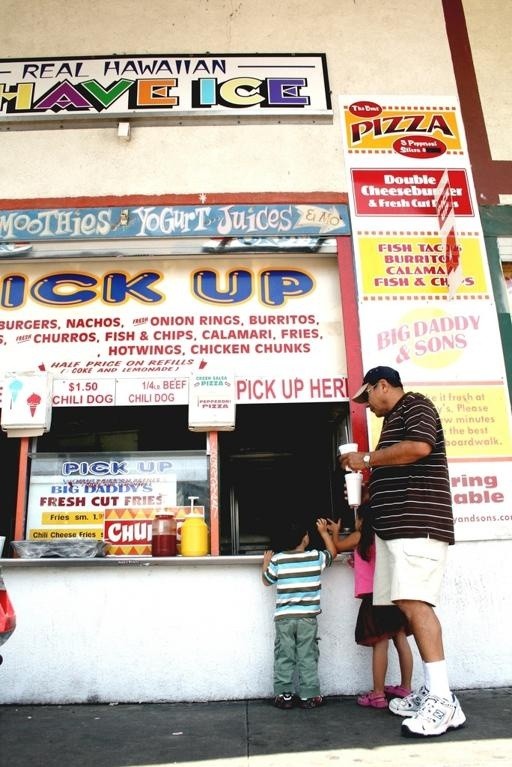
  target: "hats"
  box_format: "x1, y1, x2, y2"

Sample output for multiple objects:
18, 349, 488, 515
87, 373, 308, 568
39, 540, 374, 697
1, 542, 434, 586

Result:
351, 367, 403, 403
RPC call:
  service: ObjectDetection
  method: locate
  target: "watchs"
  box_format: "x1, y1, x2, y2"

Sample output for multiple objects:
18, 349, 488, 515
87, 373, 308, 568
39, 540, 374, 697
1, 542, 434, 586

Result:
363, 453, 371, 468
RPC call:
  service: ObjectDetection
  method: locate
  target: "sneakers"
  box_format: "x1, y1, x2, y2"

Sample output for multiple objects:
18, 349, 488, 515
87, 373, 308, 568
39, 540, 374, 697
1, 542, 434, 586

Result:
274, 695, 323, 710
357, 686, 466, 737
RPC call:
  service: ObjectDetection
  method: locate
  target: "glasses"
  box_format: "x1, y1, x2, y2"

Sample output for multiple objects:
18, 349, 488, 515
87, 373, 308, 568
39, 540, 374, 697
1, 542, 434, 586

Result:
363, 383, 378, 401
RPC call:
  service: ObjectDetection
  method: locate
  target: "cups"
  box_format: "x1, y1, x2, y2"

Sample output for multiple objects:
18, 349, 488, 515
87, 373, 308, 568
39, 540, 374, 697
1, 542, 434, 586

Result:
344, 473, 365, 505
337, 442, 357, 471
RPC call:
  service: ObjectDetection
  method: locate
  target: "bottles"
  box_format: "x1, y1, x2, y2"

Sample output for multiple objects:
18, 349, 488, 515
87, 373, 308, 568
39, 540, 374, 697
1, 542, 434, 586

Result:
151, 512, 208, 557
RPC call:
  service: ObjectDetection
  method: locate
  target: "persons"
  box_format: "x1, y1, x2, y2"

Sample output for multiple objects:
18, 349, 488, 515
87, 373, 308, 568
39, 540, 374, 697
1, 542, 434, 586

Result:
262, 518, 336, 709
338, 367, 467, 737
327, 502, 414, 708
